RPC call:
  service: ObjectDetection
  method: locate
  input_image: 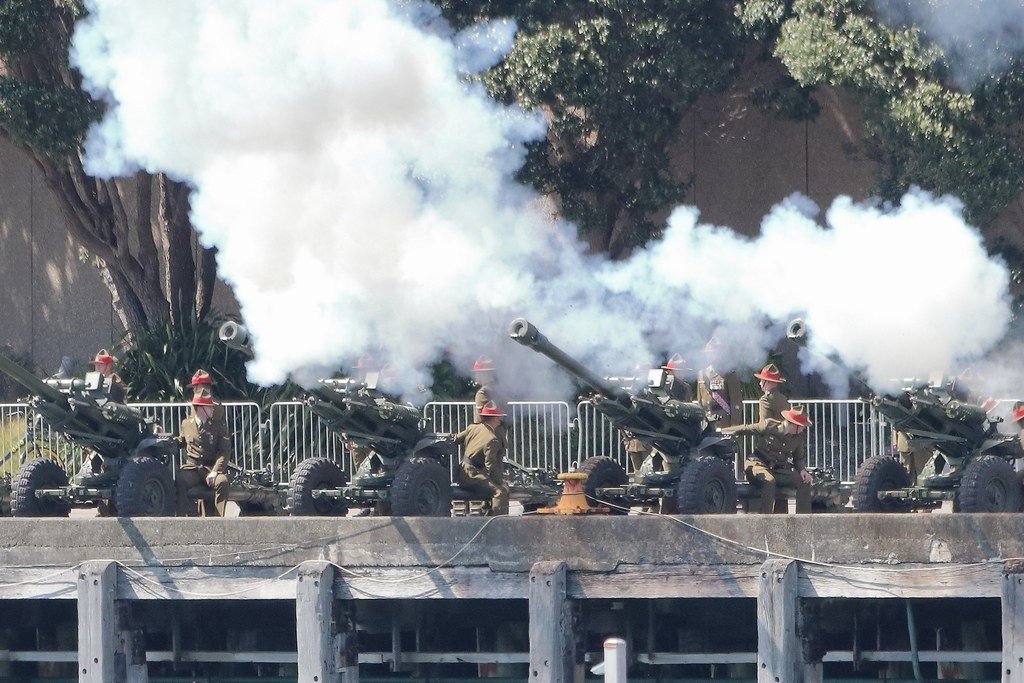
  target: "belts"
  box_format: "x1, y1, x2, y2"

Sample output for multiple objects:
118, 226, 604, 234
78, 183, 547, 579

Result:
461, 455, 485, 471
753, 449, 783, 470
186, 454, 215, 467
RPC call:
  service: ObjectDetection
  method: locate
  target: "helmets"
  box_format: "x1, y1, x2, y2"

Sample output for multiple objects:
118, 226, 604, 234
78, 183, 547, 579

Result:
1011, 401, 1024, 423
186, 370, 219, 386
88, 348, 118, 364
662, 353, 692, 371
480, 399, 506, 416
472, 354, 495, 372
754, 363, 787, 384
780, 406, 813, 426
352, 354, 375, 367
189, 389, 220, 406
981, 397, 999, 414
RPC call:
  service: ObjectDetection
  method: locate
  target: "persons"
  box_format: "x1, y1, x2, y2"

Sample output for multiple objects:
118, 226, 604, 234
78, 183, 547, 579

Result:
893, 368, 1024, 514
89, 349, 121, 385
186, 369, 217, 395
173, 388, 231, 517
754, 363, 791, 423
453, 400, 509, 516
697, 337, 743, 452
628, 353, 693, 515
472, 355, 506, 473
715, 405, 813, 514
